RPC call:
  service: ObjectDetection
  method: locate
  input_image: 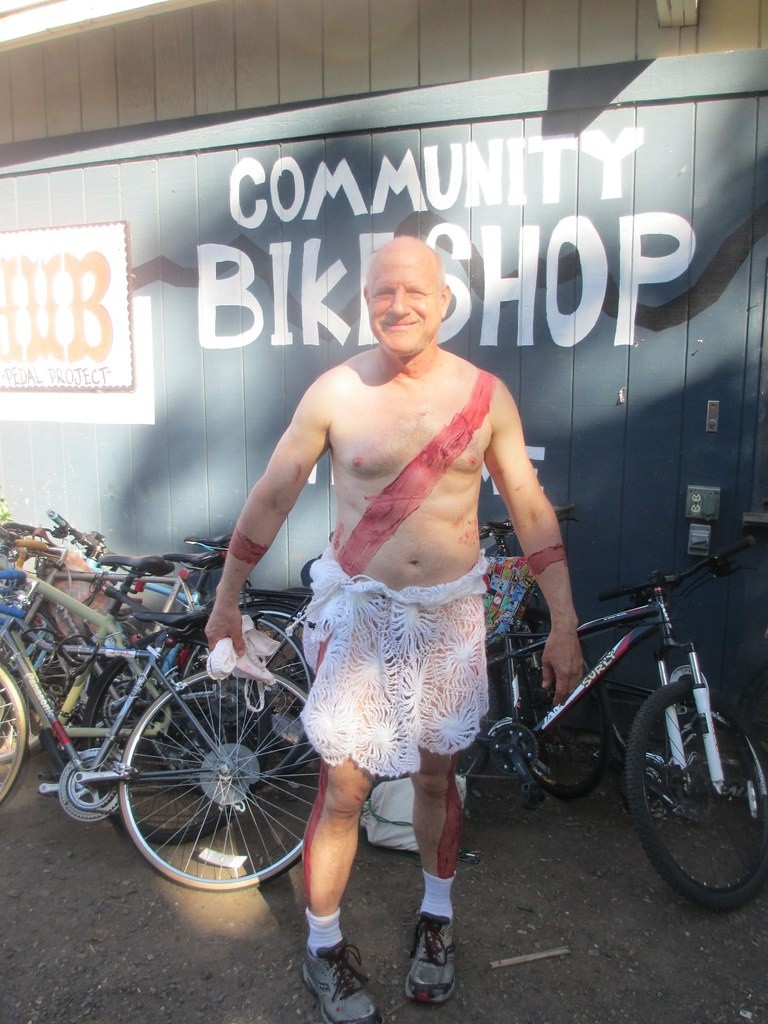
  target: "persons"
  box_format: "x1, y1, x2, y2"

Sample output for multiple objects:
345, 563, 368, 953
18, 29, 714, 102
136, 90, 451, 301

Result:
203, 238, 586, 1024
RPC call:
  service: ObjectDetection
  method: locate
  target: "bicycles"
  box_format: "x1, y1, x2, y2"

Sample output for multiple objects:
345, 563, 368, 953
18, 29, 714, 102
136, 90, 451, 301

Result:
0, 507, 767, 915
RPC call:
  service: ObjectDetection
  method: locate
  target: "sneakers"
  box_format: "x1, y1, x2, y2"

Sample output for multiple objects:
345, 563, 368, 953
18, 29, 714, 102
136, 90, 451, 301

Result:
303, 934, 379, 1022
406, 906, 456, 1004
